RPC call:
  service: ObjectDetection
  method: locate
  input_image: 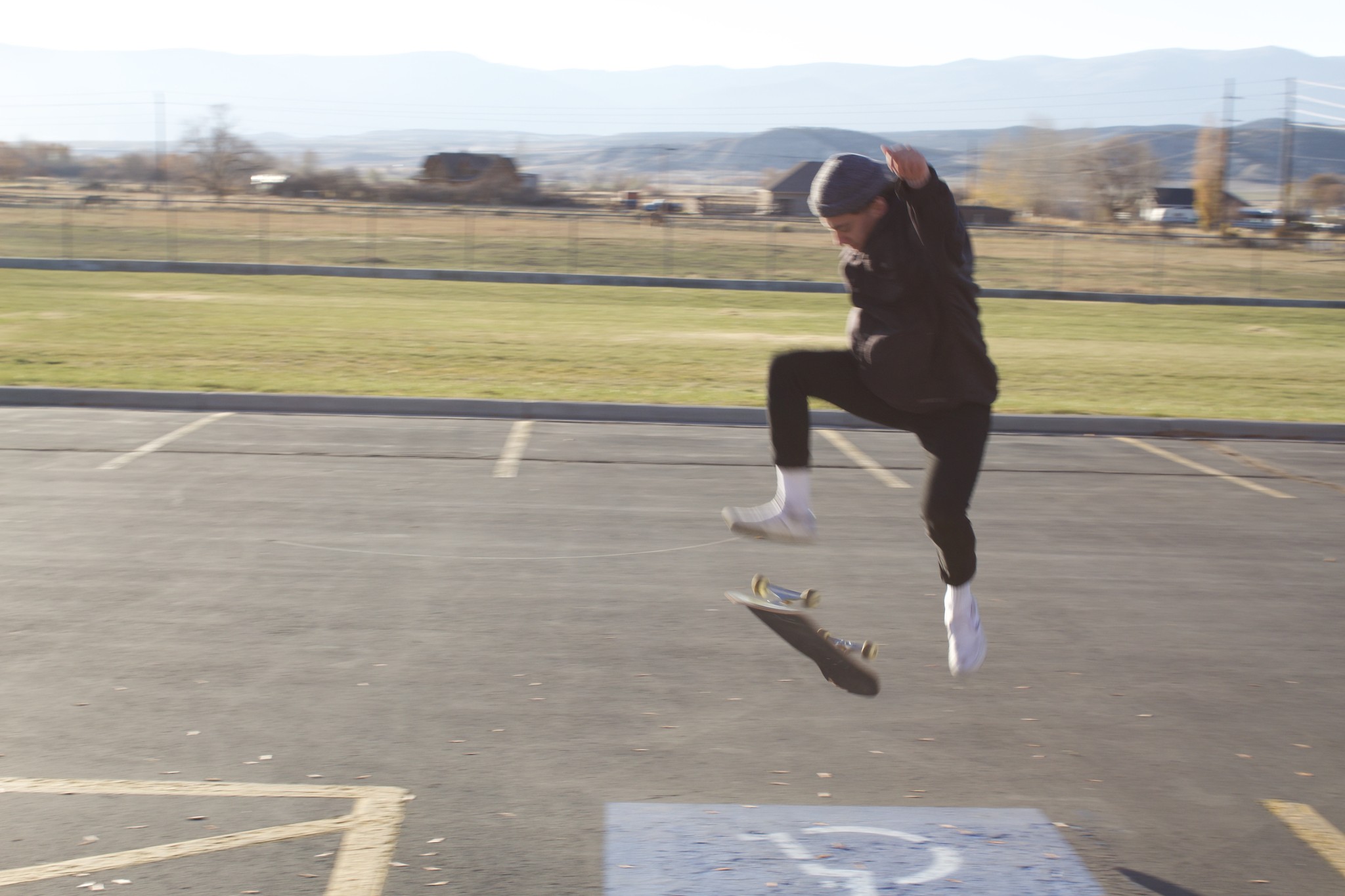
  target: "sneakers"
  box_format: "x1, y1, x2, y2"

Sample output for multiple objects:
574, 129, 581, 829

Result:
724, 500, 816, 546
945, 593, 986, 676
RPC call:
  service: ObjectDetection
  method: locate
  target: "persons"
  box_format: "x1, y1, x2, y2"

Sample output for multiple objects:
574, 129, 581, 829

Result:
720, 143, 1004, 680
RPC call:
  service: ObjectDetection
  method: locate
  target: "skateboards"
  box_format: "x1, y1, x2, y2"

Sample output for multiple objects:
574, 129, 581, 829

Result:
726, 574, 883, 697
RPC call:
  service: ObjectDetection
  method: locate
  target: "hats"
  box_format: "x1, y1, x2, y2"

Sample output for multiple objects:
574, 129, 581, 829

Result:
807, 150, 890, 216
